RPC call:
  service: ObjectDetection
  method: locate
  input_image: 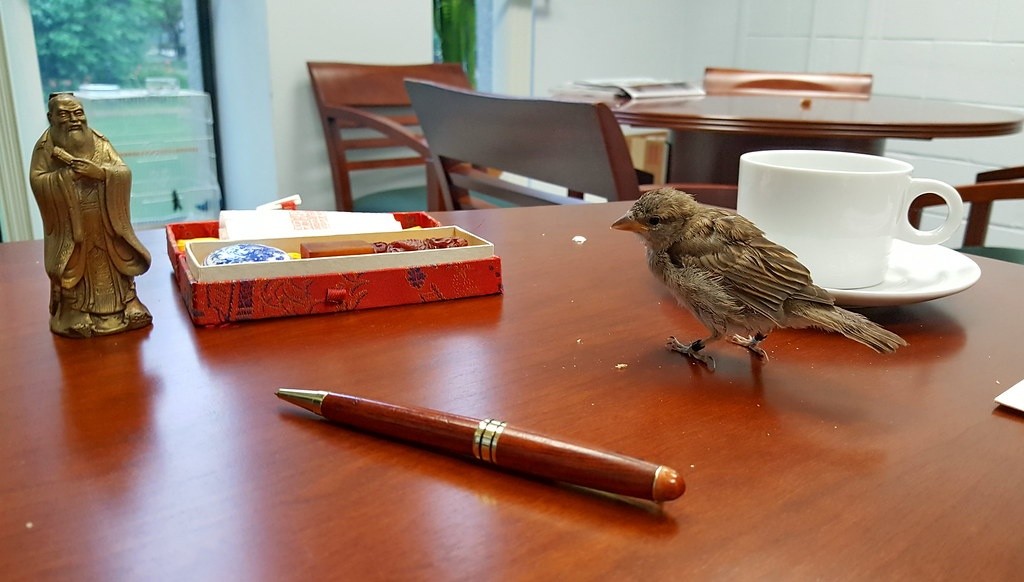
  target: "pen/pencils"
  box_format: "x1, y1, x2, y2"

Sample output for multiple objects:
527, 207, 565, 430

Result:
274, 388, 687, 505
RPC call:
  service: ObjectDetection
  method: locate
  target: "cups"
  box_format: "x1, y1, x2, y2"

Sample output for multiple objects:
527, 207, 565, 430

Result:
737, 151, 963, 288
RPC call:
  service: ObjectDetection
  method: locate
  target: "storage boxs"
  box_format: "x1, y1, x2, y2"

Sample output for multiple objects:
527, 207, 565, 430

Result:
164, 211, 443, 278
178, 254, 506, 327
185, 223, 495, 284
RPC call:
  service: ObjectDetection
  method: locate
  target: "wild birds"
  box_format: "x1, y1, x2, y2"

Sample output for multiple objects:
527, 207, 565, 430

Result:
611, 187, 911, 372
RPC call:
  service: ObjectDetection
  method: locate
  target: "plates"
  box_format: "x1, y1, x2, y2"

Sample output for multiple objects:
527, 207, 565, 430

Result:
736, 224, 982, 308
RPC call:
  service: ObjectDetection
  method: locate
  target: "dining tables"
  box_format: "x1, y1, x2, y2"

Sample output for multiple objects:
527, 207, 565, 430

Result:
539, 92, 1024, 185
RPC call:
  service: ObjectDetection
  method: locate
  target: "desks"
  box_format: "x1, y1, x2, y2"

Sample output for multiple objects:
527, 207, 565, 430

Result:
0, 197, 1024, 582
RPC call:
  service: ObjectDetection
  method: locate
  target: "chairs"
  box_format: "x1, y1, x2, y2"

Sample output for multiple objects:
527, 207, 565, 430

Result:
307, 61, 476, 213
403, 76, 737, 213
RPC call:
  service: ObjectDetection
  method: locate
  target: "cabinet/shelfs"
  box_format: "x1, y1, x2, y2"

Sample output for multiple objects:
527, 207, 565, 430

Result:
72, 87, 222, 230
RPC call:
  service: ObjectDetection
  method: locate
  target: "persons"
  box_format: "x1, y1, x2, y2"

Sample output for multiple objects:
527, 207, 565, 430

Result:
30, 92, 155, 339
